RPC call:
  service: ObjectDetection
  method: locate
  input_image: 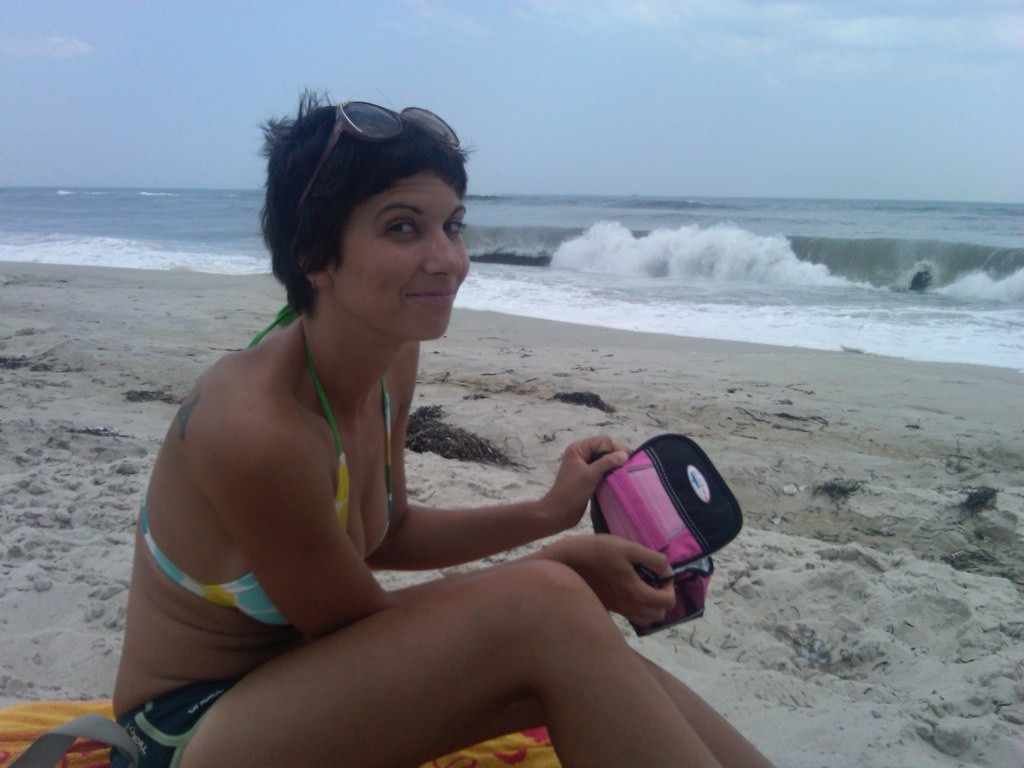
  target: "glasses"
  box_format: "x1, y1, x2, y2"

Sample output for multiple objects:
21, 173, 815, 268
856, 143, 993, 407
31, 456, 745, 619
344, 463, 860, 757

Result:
295, 100, 459, 213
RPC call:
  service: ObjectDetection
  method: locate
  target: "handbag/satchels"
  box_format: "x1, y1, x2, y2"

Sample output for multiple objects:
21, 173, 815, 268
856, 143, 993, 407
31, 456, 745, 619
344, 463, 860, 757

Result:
589, 434, 744, 637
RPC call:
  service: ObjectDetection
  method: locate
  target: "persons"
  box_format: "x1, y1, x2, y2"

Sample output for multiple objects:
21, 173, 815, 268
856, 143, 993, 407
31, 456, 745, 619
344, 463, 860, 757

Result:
112, 88, 775, 768
